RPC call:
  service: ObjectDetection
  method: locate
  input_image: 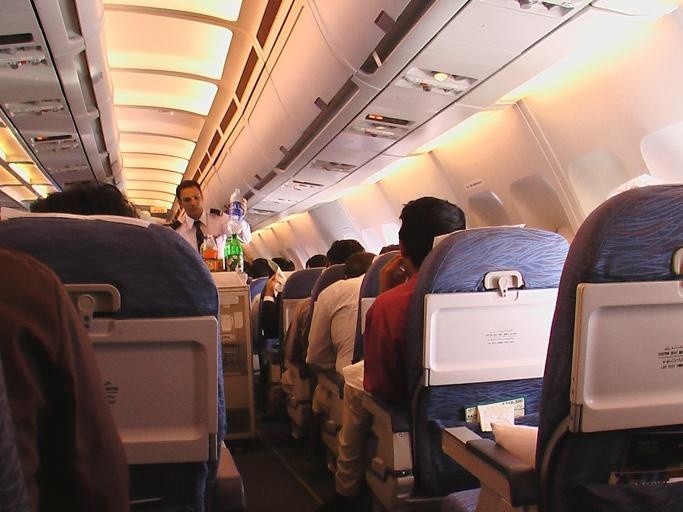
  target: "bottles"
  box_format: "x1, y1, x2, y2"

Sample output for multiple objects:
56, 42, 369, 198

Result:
224, 233, 243, 272
228, 188, 243, 233
199, 234, 218, 272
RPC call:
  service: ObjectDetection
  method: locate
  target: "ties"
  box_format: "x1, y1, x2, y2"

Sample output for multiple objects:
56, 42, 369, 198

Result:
194, 221, 205, 251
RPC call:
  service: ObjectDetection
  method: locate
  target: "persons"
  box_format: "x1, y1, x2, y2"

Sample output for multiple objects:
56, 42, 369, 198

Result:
317, 196, 467, 512
29, 181, 140, 220
242, 239, 399, 485
161, 178, 252, 270
